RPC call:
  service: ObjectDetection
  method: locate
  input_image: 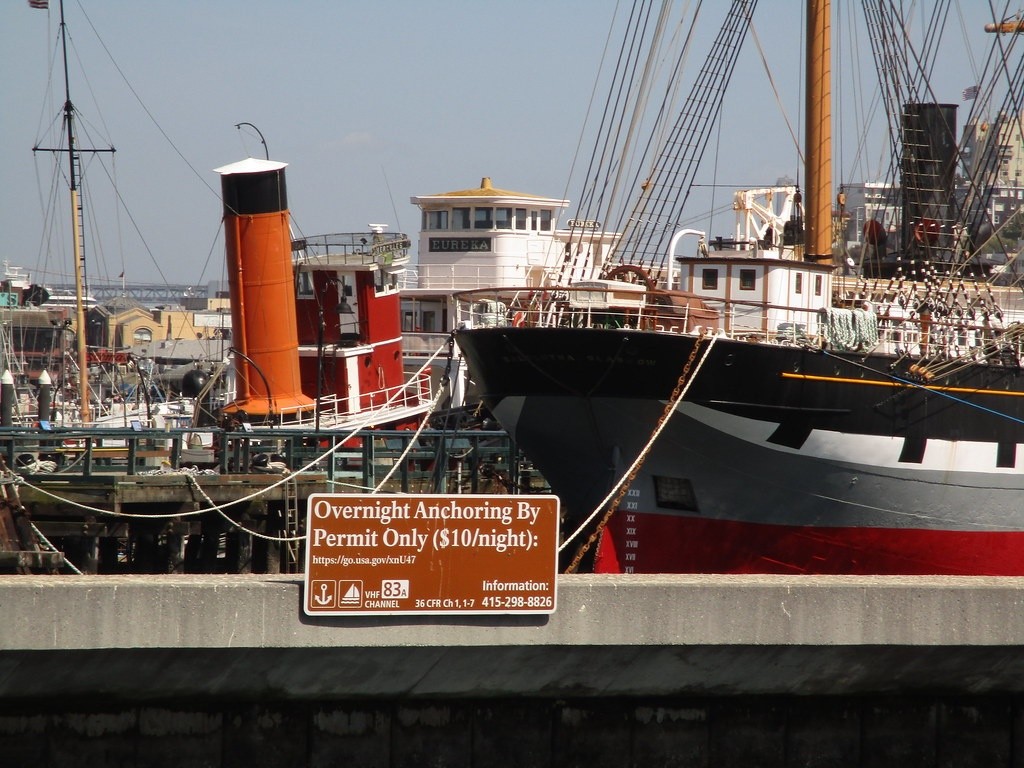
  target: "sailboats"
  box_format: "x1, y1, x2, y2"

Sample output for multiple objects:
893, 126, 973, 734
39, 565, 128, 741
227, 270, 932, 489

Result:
452, 0, 1024, 575
0, 0, 430, 473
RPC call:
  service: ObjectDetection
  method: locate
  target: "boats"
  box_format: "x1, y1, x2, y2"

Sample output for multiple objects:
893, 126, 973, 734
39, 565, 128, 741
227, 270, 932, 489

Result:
398, 174, 679, 415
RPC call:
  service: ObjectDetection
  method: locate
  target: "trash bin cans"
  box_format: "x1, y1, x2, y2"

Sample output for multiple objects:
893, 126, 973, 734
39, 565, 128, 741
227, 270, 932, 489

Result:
777, 323, 806, 343
